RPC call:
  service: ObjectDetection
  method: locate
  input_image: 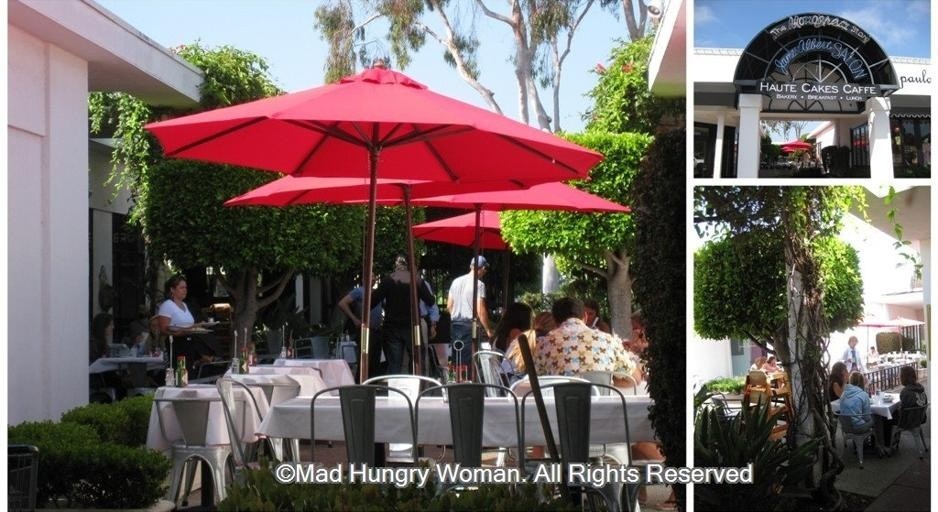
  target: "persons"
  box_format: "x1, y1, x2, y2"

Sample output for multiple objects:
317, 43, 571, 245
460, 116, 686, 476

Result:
420, 279, 441, 374
843, 336, 863, 379
159, 272, 207, 377
827, 362, 849, 402
88, 314, 129, 402
750, 356, 767, 378
839, 371, 879, 457
370, 255, 435, 374
883, 366, 927, 451
337, 271, 385, 384
446, 255, 495, 381
802, 150, 810, 169
490, 301, 546, 459
870, 346, 881, 365
764, 357, 779, 372
583, 300, 612, 335
526, 297, 642, 396
922, 137, 930, 167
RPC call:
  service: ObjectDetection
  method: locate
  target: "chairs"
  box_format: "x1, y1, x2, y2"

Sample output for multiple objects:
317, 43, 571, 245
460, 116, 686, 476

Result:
154, 398, 247, 509
217, 374, 284, 473
472, 351, 515, 397
360, 374, 446, 464
549, 369, 636, 396
891, 402, 930, 461
746, 369, 752, 375
294, 336, 340, 358
521, 381, 648, 512
310, 386, 416, 470
714, 391, 739, 418
832, 410, 886, 468
415, 382, 520, 473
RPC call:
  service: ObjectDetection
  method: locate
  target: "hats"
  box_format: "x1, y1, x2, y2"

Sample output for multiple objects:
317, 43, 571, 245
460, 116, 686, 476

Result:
471, 255, 489, 268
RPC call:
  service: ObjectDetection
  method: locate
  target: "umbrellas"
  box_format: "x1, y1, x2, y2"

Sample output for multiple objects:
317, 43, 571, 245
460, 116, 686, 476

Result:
223, 173, 543, 457
779, 138, 812, 154
346, 181, 632, 383
144, 66, 606, 384
411, 210, 513, 316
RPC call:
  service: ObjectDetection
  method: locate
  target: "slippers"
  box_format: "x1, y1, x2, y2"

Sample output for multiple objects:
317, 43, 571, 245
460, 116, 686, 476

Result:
656, 500, 677, 510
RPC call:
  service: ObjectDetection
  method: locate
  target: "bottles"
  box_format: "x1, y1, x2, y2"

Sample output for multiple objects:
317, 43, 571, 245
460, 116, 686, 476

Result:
176, 356, 185, 387
460, 364, 469, 383
286, 340, 294, 359
181, 356, 189, 385
249, 343, 257, 366
244, 348, 249, 373
448, 365, 455, 383
240, 348, 246, 373
